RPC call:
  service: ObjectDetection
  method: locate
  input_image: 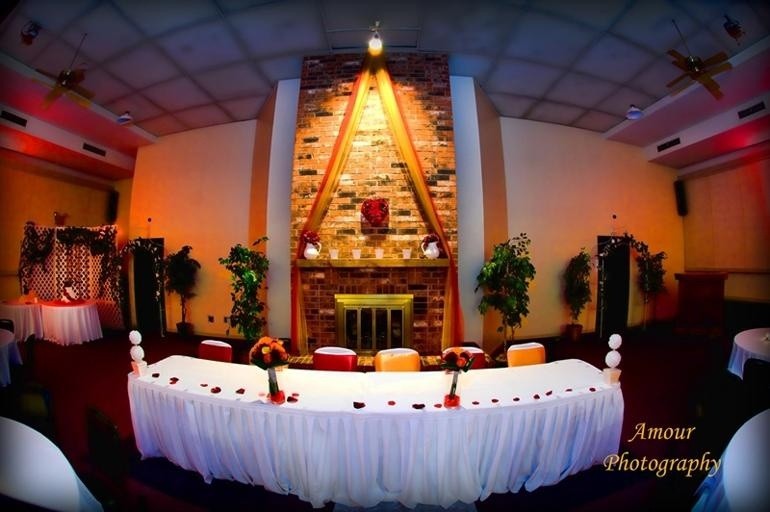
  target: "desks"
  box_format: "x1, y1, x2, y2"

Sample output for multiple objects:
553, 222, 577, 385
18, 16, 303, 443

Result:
0, 416, 103, 511
126, 353, 624, 510
1, 326, 24, 385
37, 297, 104, 346
692, 408, 769, 512
726, 326, 770, 380
0, 296, 44, 342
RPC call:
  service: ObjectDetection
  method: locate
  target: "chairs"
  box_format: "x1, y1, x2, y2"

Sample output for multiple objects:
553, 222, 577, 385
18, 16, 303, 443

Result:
736, 357, 769, 425
443, 346, 487, 370
373, 347, 423, 373
73, 401, 128, 511
506, 342, 545, 368
195, 338, 234, 362
313, 345, 359, 373
12, 380, 63, 447
8, 332, 37, 387
1, 317, 14, 335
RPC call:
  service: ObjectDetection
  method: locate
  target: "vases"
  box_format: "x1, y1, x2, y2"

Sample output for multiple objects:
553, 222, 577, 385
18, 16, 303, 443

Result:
303, 241, 322, 261
447, 369, 458, 398
420, 241, 441, 259
267, 367, 279, 397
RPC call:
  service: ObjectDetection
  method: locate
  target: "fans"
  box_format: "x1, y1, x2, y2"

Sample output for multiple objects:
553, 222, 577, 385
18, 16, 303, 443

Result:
659, 14, 734, 100
37, 29, 96, 109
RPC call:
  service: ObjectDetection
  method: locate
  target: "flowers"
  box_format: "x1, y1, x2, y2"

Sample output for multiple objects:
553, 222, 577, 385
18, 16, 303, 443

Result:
107, 236, 167, 308
301, 229, 322, 244
439, 350, 473, 375
422, 232, 439, 246
250, 334, 291, 369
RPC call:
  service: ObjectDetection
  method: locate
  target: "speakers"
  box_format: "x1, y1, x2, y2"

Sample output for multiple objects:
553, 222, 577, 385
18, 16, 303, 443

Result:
133, 237, 167, 337
672, 180, 688, 217
596, 235, 630, 344
105, 190, 119, 223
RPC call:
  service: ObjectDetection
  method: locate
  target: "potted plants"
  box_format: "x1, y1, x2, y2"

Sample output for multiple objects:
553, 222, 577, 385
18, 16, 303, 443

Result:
161, 240, 199, 337
557, 247, 592, 345
473, 232, 537, 368
